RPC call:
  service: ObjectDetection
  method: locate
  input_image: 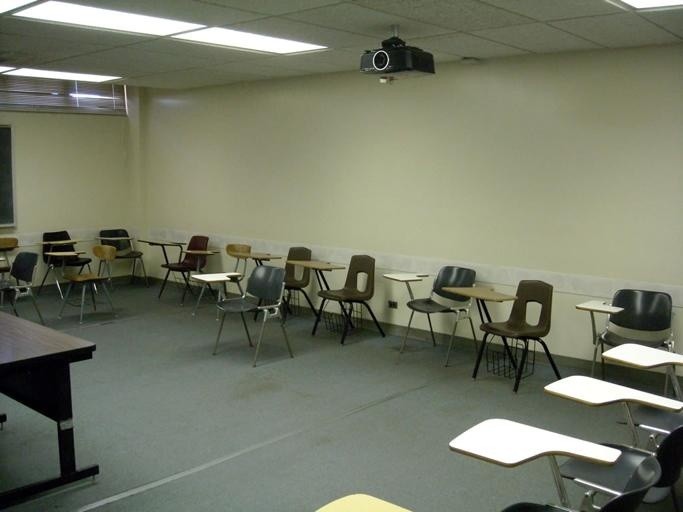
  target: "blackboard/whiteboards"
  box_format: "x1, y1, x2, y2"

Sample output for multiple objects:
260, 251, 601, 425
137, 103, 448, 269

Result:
0, 123, 17, 227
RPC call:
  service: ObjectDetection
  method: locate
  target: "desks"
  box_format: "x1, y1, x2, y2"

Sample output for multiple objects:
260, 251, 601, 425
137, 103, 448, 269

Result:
314, 493, 412, 512
1, 311, 101, 512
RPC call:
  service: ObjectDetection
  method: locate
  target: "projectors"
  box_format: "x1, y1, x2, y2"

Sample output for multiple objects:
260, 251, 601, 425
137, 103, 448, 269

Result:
360, 46, 435, 79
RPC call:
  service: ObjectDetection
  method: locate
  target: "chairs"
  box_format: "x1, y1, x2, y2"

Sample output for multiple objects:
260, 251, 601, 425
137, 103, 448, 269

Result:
35, 231, 99, 301
449, 418, 662, 512
544, 375, 683, 511
573, 288, 683, 411
45, 245, 120, 323
386, 267, 482, 368
94, 229, 151, 289
182, 244, 251, 321
286, 254, 383, 344
229, 245, 321, 322
0, 252, 44, 325
138, 235, 216, 304
441, 278, 562, 395
191, 265, 294, 367
1, 237, 41, 303
